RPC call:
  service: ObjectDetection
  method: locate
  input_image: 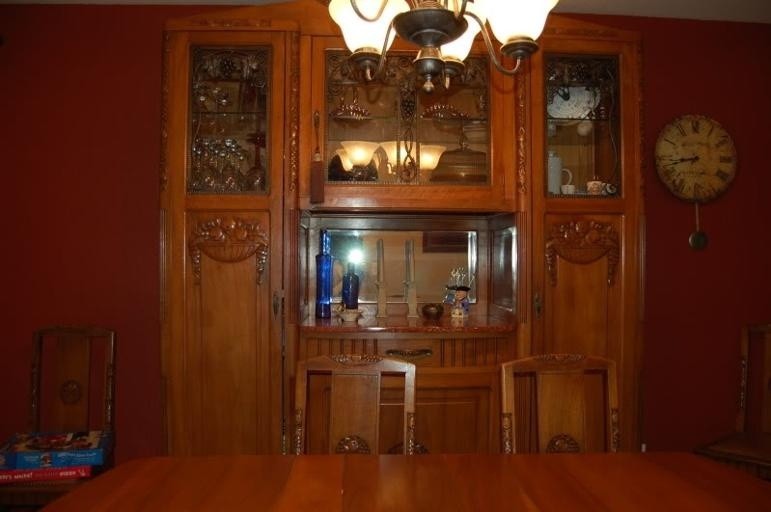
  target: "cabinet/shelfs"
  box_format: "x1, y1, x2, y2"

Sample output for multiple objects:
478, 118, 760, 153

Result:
159, 3, 641, 452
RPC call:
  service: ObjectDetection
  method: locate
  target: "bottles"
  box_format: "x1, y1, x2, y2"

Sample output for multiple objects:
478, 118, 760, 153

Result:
343, 262, 358, 307
315, 230, 333, 318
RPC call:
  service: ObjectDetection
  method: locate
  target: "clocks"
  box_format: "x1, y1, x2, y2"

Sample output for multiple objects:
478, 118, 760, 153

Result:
655, 114, 738, 248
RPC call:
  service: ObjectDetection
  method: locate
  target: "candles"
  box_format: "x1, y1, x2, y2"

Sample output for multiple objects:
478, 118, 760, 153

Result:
376, 236, 416, 283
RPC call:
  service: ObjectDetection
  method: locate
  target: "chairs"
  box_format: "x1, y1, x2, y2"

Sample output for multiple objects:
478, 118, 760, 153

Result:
498, 355, 621, 455
694, 323, 770, 476
0, 325, 115, 506
293, 354, 419, 454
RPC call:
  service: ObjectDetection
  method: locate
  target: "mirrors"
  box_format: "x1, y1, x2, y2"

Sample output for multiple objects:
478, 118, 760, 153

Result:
324, 228, 478, 306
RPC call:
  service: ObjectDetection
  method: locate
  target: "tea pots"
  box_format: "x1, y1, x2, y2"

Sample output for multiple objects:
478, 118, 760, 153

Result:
547, 150, 572, 192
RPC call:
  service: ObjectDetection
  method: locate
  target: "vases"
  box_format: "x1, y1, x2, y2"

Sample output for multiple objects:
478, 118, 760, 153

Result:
315, 228, 359, 321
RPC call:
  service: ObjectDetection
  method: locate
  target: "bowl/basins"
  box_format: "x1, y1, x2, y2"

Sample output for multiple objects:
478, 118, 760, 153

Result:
338, 310, 362, 322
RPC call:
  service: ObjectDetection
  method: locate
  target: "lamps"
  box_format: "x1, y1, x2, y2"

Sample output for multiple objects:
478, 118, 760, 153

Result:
326, 0, 559, 96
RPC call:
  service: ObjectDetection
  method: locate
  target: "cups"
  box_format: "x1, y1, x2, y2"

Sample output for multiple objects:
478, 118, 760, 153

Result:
561, 184, 575, 194
588, 181, 606, 193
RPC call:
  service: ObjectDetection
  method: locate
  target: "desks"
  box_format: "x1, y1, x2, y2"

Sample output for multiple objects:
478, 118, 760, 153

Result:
36, 452, 771, 511
